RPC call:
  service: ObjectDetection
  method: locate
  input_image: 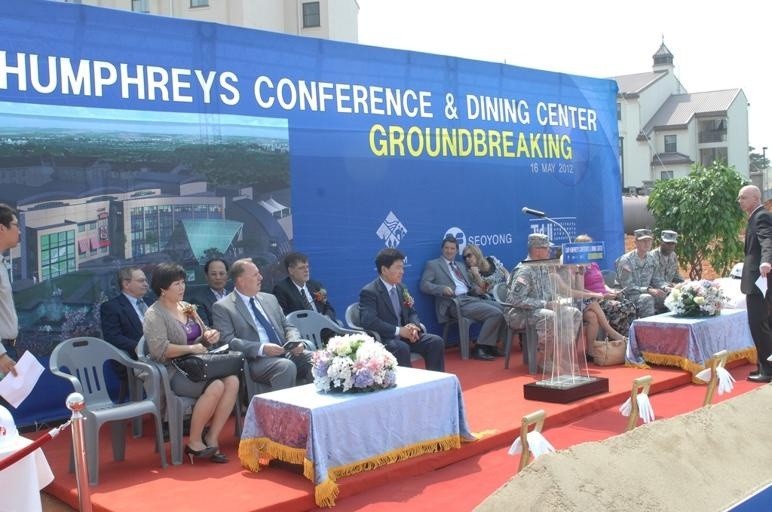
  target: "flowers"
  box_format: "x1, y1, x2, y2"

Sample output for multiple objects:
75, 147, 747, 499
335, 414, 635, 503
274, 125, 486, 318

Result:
308, 333, 397, 393
664, 278, 732, 316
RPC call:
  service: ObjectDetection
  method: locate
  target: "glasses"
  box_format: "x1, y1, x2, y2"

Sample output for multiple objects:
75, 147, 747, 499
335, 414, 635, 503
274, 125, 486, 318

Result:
464, 253, 474, 260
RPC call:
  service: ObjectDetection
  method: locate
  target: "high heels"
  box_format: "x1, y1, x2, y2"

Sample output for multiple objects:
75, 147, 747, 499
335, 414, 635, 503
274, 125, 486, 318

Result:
185, 437, 228, 464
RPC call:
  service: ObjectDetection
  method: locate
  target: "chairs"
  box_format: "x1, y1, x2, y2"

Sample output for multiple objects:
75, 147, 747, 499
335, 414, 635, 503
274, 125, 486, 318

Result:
286, 310, 367, 351
443, 292, 491, 359
49, 336, 169, 487
493, 283, 539, 375
229, 351, 307, 436
135, 335, 242, 465
345, 302, 428, 362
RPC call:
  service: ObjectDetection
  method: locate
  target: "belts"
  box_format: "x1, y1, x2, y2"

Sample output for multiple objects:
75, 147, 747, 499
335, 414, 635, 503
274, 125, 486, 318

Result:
1, 338, 16, 348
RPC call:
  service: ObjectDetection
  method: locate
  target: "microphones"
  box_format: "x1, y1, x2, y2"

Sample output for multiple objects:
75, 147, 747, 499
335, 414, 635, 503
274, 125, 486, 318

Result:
522, 206, 547, 218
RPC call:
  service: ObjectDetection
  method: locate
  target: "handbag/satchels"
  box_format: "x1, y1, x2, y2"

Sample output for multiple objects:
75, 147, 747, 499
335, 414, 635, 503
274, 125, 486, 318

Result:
171, 344, 246, 382
592, 336, 628, 366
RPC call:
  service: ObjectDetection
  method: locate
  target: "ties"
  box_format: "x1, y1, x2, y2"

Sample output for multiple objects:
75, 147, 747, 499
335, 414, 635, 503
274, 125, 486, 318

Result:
249, 298, 282, 347
135, 299, 148, 315
450, 262, 464, 279
390, 287, 401, 325
301, 287, 314, 310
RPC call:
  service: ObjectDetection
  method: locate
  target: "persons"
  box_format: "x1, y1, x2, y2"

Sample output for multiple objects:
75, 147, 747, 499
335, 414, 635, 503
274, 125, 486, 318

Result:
142, 263, 239, 466
0, 203, 21, 375
210, 258, 315, 388
557, 253, 628, 365
574, 235, 637, 340
101, 265, 191, 442
420, 237, 506, 359
503, 232, 583, 375
619, 229, 670, 320
651, 231, 687, 311
192, 258, 230, 328
273, 253, 336, 346
359, 250, 445, 371
462, 245, 511, 291
738, 186, 772, 381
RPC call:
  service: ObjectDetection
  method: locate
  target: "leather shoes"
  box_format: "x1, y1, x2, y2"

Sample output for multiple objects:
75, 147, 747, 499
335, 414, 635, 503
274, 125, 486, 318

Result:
472, 344, 506, 360
748, 367, 772, 381
161, 419, 190, 441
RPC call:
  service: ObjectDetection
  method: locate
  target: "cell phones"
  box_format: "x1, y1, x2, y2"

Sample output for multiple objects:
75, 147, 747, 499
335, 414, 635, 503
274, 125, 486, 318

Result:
615, 287, 627, 295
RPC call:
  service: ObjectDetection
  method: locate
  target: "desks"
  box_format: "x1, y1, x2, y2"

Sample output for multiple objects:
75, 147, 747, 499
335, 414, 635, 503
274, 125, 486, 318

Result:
238, 366, 494, 508
624, 309, 758, 385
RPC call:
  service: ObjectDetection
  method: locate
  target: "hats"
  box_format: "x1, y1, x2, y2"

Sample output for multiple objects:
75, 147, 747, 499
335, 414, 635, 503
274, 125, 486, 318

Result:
634, 229, 655, 240
527, 234, 555, 248
661, 230, 678, 245
